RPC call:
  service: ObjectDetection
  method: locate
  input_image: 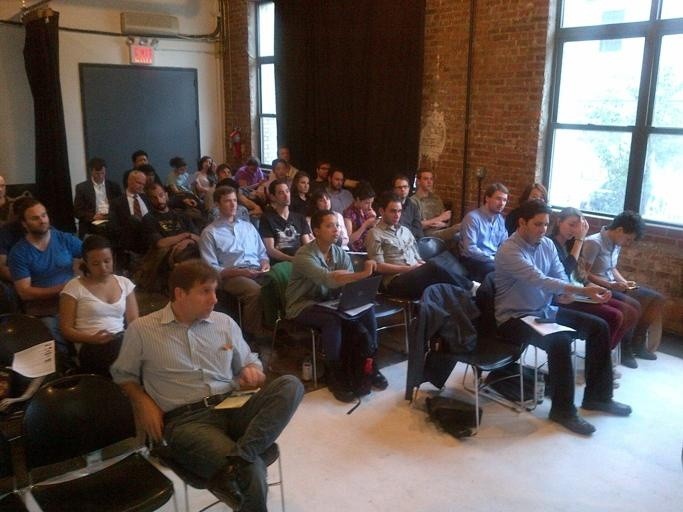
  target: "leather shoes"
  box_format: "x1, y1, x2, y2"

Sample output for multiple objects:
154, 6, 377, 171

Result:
369, 362, 389, 390
208, 468, 244, 505
327, 374, 355, 403
549, 412, 596, 433
582, 399, 631, 416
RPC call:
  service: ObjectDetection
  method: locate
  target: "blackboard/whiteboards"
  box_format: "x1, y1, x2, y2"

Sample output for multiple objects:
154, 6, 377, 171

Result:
78, 63, 201, 186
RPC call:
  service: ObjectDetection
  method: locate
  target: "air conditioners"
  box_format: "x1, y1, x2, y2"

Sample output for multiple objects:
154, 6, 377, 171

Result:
119, 9, 181, 39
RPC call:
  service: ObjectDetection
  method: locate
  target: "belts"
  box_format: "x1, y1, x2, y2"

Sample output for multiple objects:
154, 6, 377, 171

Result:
166, 394, 225, 420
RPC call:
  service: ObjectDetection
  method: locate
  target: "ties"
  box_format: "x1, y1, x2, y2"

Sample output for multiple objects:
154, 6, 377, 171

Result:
133, 194, 142, 217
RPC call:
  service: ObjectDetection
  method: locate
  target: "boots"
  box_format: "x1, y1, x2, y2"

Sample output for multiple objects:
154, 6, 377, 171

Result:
632, 335, 656, 360
620, 337, 638, 370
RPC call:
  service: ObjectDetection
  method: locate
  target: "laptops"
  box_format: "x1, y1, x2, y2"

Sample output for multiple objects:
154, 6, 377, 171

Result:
319, 272, 385, 314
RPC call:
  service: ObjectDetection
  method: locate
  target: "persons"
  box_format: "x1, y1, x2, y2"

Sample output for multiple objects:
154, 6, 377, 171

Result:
283, 208, 390, 403
0, 144, 667, 390
107, 258, 306, 511
493, 197, 633, 436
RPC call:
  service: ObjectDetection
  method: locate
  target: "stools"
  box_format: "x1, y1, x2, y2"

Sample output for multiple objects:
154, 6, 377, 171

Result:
78, 219, 95, 243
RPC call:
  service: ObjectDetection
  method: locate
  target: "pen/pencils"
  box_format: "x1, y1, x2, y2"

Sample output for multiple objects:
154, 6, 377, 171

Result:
415, 256, 421, 264
228, 392, 254, 397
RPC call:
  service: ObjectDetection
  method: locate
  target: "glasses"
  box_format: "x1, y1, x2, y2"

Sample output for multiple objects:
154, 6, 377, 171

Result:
394, 185, 410, 190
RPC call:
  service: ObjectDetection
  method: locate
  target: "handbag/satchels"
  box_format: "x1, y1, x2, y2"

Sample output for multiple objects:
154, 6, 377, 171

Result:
341, 335, 373, 415
485, 362, 549, 400
424, 396, 482, 438
429, 250, 469, 278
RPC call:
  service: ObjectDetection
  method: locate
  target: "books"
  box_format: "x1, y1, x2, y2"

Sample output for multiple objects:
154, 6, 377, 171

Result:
214, 387, 260, 409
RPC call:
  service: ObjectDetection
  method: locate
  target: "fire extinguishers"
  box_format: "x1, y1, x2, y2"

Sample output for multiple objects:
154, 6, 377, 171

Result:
230, 127, 241, 159
